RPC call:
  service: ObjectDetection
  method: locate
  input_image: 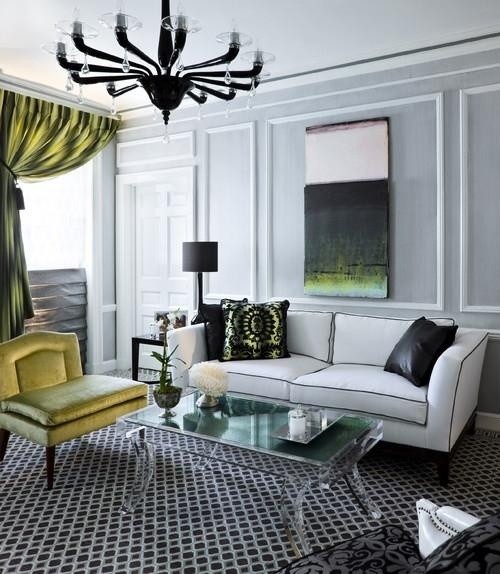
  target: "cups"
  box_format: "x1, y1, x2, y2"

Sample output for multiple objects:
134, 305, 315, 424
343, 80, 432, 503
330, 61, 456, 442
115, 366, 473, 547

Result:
286, 406, 329, 441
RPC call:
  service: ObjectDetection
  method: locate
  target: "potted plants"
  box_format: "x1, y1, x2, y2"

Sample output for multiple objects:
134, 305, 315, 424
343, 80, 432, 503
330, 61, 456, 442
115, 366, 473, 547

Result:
150, 333, 185, 408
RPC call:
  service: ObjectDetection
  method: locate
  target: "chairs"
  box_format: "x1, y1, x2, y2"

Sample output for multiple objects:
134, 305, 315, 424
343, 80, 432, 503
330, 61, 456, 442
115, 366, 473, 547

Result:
269, 498, 500, 573
1, 331, 144, 490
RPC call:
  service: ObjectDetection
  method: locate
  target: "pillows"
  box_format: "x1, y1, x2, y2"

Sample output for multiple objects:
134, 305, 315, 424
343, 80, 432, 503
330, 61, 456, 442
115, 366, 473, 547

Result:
223, 298, 290, 359
383, 313, 459, 384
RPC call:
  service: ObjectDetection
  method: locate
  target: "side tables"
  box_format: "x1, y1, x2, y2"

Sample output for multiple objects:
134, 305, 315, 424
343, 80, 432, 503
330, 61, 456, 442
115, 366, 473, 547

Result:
131, 334, 166, 381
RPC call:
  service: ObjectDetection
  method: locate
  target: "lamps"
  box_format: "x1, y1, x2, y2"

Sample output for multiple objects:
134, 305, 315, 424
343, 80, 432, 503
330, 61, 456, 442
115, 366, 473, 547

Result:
183, 240, 217, 323
42, 1, 276, 147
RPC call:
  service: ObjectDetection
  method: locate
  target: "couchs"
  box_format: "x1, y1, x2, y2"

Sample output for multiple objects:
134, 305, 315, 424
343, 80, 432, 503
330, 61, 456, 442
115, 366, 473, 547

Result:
170, 311, 488, 487
